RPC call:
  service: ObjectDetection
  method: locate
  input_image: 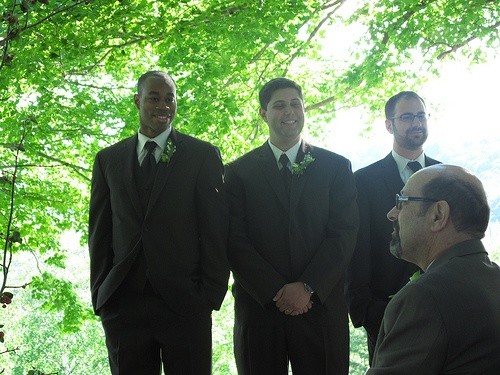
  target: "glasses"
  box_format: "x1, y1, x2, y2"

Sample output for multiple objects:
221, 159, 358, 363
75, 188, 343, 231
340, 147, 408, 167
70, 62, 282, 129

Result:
390, 112, 430, 121
396, 193, 440, 210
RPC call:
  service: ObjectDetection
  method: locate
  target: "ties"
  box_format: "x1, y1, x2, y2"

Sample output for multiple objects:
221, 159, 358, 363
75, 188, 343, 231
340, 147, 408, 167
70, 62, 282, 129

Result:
136, 140, 159, 201
279, 154, 292, 193
407, 161, 422, 173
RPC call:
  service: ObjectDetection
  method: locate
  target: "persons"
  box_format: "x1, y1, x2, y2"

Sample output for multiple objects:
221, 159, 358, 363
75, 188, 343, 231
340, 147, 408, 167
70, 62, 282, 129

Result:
366, 164, 500, 375
88, 71, 230, 375
349, 91, 443, 366
224, 78, 360, 375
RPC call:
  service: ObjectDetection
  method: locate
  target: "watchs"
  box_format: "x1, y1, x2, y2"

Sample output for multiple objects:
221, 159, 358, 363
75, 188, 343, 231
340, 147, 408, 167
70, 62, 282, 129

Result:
304, 283, 314, 294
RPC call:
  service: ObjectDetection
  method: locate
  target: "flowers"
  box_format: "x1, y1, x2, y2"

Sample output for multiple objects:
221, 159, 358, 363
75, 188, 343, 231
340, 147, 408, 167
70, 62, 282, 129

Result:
290, 152, 316, 176
389, 270, 422, 299
161, 137, 176, 167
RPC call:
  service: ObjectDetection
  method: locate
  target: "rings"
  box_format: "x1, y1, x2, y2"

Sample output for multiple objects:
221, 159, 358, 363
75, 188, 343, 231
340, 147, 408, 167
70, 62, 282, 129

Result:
287, 309, 289, 314
